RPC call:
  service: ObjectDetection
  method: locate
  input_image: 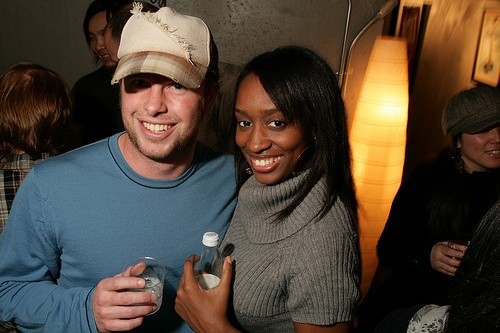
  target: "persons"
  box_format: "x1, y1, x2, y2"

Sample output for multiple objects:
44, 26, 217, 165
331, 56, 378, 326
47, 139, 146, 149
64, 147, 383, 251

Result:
0, 3, 253, 333
175, 46, 361, 333
0, 0, 243, 236
356, 85, 500, 333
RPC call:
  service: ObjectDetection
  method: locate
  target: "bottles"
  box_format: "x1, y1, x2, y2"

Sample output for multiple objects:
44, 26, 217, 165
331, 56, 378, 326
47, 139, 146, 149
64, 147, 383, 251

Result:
192, 232, 224, 291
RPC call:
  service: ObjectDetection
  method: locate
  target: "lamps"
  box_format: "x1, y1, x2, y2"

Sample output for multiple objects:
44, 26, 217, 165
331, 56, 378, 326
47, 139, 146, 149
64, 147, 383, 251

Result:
339, 35, 410, 289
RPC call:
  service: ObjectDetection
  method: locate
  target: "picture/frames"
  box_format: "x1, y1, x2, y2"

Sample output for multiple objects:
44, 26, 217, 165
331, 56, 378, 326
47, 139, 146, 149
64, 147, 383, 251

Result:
471, 9, 500, 88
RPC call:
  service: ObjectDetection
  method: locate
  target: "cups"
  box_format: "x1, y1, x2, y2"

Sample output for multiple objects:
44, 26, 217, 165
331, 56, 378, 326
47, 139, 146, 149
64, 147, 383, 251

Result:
121, 256, 164, 315
447, 240, 473, 269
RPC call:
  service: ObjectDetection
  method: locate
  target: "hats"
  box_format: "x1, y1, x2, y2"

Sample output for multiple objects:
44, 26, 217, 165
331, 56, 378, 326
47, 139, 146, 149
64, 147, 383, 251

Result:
111, 1, 220, 90
441, 86, 500, 143
